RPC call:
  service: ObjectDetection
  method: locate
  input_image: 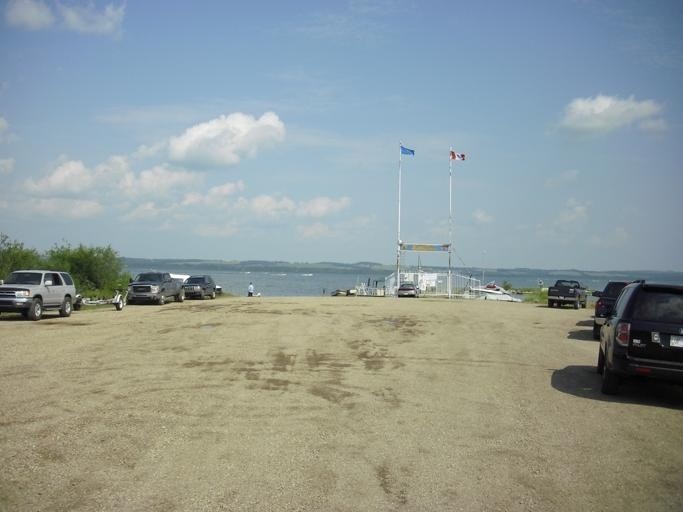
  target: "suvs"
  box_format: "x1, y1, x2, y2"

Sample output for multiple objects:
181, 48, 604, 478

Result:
0, 270, 76, 320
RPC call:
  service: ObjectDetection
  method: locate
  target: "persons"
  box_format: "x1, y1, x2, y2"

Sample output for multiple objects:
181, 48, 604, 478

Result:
247, 281, 255, 297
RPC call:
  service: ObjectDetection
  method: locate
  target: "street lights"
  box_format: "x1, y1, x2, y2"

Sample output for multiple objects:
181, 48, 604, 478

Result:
482, 250, 487, 281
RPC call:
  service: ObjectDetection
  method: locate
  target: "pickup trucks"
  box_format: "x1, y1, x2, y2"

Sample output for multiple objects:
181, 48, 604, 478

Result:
548, 280, 587, 309
128, 272, 183, 305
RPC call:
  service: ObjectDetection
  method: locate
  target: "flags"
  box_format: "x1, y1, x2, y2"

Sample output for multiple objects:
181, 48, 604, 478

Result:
450, 151, 466, 161
399, 146, 416, 157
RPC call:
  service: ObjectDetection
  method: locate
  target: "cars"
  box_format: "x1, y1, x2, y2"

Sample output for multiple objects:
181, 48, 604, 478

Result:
592, 280, 683, 395
182, 275, 216, 300
398, 284, 419, 298
